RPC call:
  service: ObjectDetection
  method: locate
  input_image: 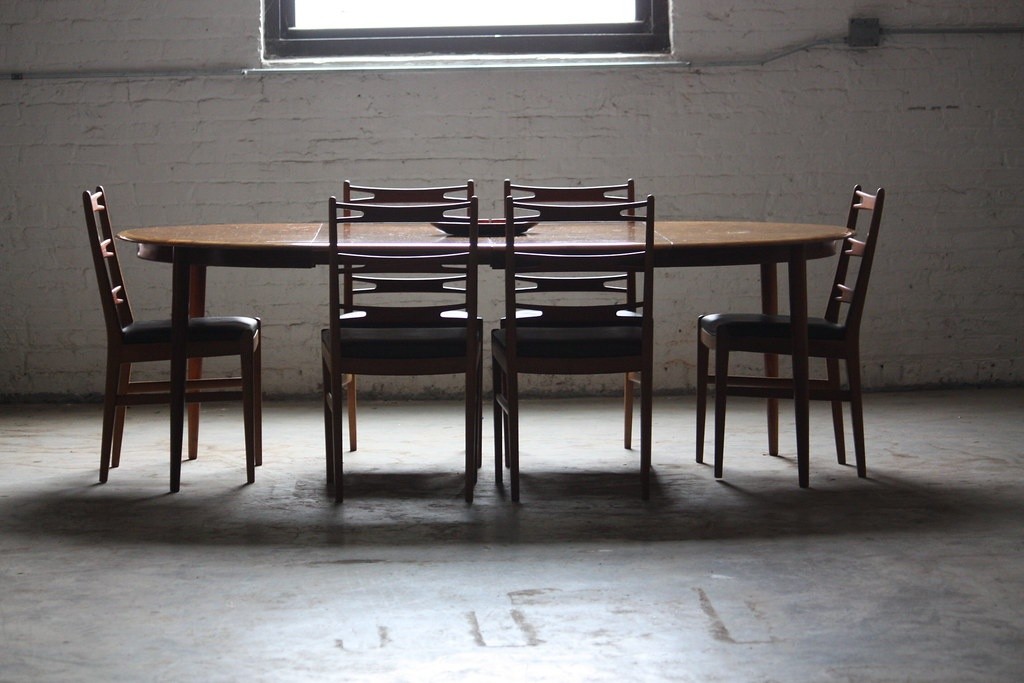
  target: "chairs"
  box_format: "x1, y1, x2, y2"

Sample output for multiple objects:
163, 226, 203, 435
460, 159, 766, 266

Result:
321, 198, 484, 504
82, 185, 262, 483
504, 180, 646, 469
695, 183, 886, 479
490, 195, 656, 502
332, 181, 481, 472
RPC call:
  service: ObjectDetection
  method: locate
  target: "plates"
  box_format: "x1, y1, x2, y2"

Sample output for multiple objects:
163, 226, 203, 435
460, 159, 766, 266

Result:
431, 222, 537, 236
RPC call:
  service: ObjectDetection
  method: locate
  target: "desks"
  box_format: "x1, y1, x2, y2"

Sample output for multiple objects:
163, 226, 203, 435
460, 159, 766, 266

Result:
115, 221, 859, 494
